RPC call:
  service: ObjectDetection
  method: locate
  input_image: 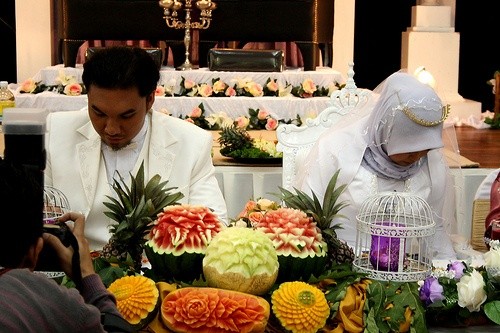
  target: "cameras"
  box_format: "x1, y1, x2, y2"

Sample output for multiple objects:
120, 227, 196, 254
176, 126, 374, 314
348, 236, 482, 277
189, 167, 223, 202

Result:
33, 223, 73, 270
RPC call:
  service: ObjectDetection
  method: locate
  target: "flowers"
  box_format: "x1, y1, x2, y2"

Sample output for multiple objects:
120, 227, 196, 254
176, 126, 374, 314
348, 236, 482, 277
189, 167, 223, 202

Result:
19, 77, 356, 132
419, 259, 500, 325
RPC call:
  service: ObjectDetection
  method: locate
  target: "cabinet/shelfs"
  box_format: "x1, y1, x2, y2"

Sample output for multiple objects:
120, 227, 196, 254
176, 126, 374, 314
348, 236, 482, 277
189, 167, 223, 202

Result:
14, 63, 375, 131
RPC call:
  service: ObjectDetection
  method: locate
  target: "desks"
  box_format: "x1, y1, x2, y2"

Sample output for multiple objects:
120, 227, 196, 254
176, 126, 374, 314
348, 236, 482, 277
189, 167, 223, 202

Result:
203, 132, 480, 219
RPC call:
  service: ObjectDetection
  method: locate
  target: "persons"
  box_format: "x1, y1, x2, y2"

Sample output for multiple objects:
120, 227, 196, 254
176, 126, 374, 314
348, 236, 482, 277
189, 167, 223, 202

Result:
484, 171, 500, 250
0, 162, 122, 333
289, 72, 457, 261
43, 45, 228, 254
